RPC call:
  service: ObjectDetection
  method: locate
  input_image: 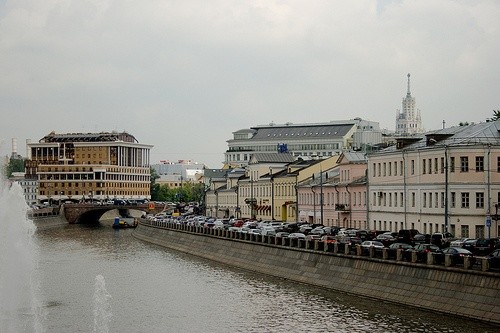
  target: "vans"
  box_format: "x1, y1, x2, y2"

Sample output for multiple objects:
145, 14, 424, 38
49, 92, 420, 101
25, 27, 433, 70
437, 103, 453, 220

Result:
430, 232, 454, 245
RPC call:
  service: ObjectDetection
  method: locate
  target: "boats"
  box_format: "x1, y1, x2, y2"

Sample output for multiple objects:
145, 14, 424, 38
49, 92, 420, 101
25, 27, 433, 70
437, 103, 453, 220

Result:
112, 222, 138, 230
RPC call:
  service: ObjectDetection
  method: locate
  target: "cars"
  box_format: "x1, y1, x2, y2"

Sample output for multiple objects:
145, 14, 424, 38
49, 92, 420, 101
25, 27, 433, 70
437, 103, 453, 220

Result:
443, 247, 472, 257
33, 198, 204, 213
149, 213, 429, 245
389, 243, 415, 259
414, 244, 442, 262
460, 239, 477, 250
476, 237, 500, 255
449, 238, 466, 247
486, 249, 500, 273
359, 241, 389, 255
